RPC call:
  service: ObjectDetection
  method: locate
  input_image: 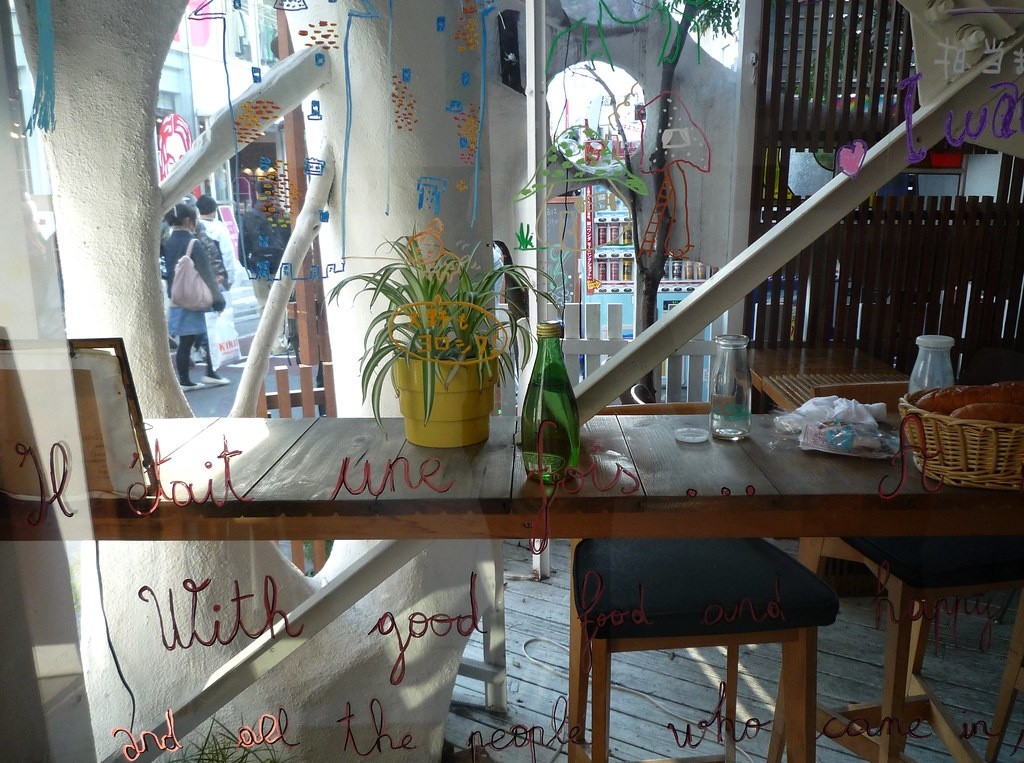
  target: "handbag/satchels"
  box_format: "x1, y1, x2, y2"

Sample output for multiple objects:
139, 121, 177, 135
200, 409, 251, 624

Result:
204, 306, 243, 372
218, 283, 231, 309
172, 238, 213, 311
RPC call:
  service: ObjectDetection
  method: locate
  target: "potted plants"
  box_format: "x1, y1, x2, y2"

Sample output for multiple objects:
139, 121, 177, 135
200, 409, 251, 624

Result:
320, 221, 562, 448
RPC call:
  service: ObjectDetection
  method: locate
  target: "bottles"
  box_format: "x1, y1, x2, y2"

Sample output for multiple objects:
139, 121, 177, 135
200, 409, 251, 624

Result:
521, 320, 580, 484
709, 334, 753, 442
908, 335, 955, 396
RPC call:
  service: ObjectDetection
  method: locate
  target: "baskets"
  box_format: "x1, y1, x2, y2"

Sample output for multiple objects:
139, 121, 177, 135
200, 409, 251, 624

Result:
899, 384, 1024, 491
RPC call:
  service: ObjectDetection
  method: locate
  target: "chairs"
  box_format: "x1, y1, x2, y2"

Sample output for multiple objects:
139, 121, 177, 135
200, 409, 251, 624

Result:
567, 401, 841, 763
765, 382, 1024, 763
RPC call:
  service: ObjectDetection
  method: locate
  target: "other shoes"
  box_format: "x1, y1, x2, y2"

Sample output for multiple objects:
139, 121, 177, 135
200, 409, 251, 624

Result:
190, 346, 206, 362
201, 376, 230, 385
177, 382, 206, 392
168, 336, 178, 350
281, 344, 294, 355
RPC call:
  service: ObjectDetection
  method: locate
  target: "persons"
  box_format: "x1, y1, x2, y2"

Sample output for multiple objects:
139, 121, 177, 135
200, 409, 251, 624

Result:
238, 179, 291, 355
160, 194, 236, 393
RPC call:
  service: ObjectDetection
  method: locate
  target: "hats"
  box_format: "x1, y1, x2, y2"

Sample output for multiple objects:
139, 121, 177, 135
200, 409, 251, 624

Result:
195, 195, 218, 215
255, 179, 276, 196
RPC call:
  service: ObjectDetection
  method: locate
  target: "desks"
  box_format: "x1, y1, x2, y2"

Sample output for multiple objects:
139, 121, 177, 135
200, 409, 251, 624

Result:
0, 418, 1024, 541
746, 348, 912, 411
